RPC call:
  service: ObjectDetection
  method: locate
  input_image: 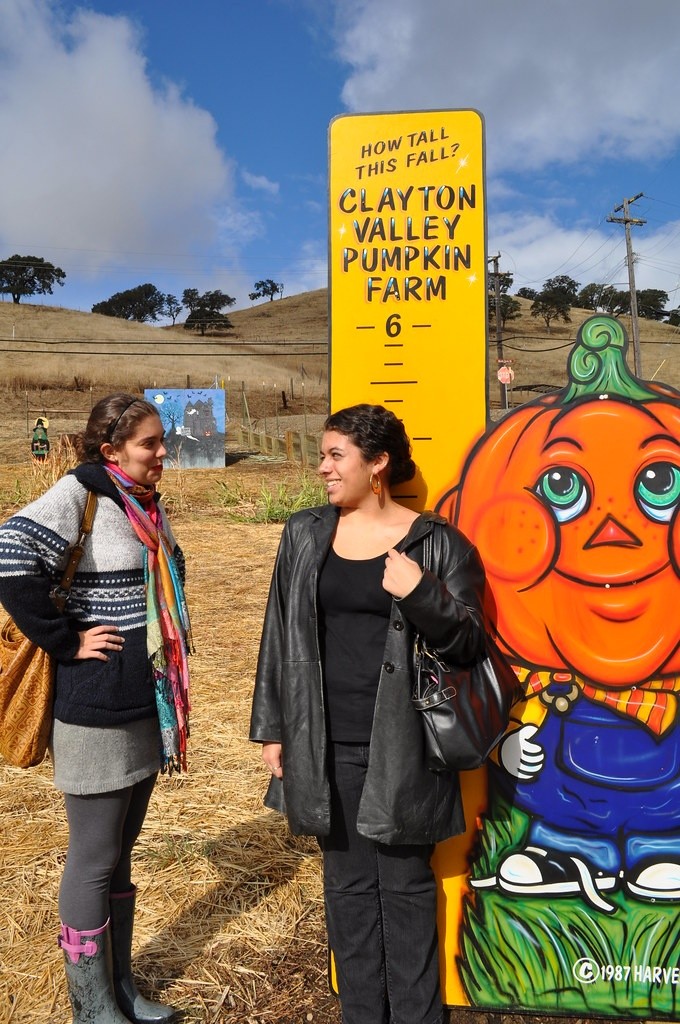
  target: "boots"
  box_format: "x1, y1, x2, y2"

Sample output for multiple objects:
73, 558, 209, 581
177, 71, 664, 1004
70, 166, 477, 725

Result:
60, 885, 173, 1023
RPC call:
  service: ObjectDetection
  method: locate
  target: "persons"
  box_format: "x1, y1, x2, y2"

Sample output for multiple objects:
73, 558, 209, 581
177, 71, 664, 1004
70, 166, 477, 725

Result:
2, 389, 201, 1023
248, 401, 500, 1024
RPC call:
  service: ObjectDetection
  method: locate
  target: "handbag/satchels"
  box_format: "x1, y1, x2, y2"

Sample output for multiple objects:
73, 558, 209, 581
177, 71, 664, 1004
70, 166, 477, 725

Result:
0, 615, 54, 769
413, 633, 527, 771
